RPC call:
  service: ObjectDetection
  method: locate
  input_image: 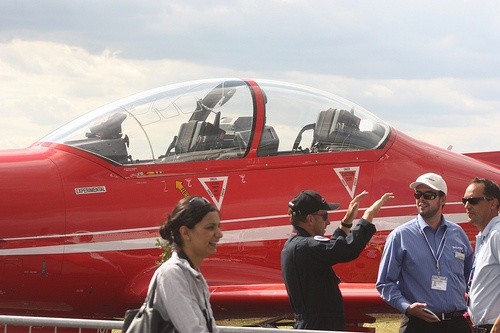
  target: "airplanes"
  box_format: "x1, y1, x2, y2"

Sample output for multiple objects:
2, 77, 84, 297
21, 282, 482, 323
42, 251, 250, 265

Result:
0, 76, 500, 333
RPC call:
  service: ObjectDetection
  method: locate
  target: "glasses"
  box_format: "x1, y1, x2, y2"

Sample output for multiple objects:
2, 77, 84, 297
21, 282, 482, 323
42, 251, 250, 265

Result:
308, 212, 328, 221
462, 196, 494, 205
180, 197, 210, 220
414, 191, 445, 200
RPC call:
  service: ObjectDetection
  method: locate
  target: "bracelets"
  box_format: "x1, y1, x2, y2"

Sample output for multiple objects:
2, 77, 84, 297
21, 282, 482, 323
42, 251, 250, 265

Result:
341, 219, 353, 228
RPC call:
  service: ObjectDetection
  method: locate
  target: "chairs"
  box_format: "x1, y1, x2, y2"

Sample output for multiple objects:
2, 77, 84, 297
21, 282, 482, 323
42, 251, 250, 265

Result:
161, 120, 208, 164
314, 107, 344, 149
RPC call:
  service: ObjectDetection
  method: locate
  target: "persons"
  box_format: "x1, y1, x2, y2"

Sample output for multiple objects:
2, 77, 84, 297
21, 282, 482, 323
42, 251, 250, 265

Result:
280, 190, 395, 331
375, 172, 474, 333
146, 195, 223, 333
461, 177, 500, 333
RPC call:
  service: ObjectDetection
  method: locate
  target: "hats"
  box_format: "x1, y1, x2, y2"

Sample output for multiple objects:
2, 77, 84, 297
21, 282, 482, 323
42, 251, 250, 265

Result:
409, 173, 447, 195
288, 190, 340, 215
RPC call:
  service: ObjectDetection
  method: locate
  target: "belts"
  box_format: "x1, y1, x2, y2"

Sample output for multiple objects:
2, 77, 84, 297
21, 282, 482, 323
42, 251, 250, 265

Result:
436, 312, 463, 320
473, 325, 491, 333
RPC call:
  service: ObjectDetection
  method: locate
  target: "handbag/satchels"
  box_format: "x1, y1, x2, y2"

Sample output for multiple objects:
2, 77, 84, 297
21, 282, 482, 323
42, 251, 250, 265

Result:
121, 272, 213, 333
